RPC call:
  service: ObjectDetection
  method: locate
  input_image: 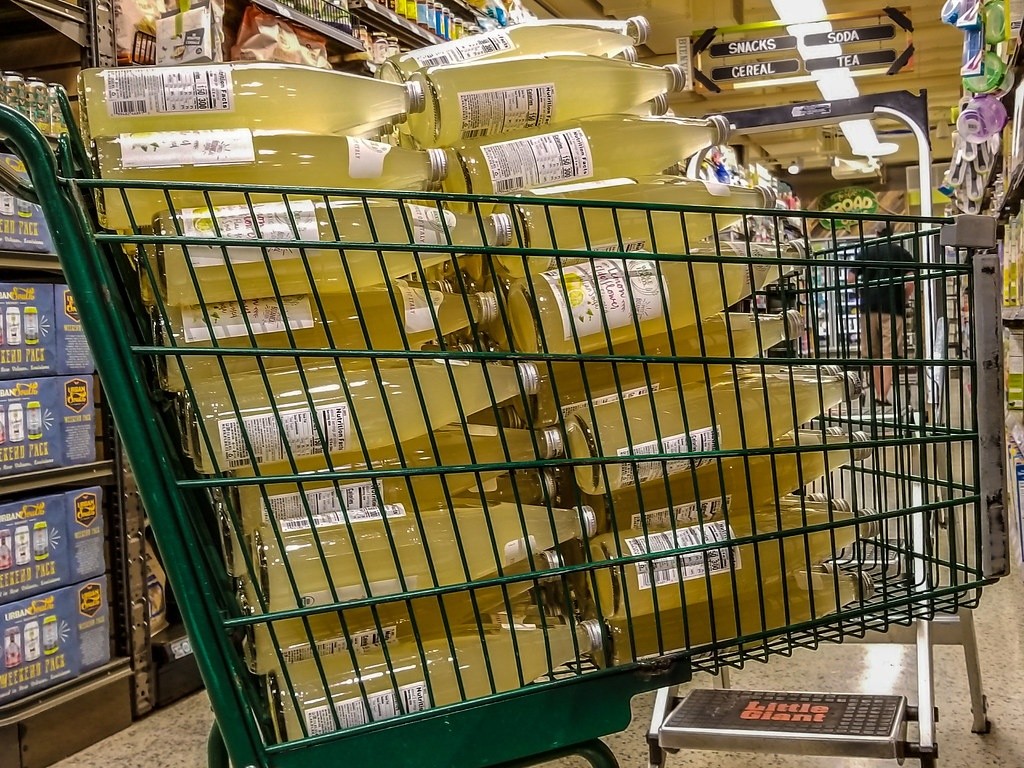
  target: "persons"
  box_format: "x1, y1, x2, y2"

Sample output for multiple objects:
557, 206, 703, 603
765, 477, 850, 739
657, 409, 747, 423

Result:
849, 220, 922, 408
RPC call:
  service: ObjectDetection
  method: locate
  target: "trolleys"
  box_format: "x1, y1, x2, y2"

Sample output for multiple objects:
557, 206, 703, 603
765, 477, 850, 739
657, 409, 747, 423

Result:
0, 88, 1013, 768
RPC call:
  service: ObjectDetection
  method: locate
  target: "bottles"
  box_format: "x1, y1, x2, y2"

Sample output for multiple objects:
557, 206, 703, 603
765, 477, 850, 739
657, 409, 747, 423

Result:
956, 0, 1014, 145
75, 15, 888, 750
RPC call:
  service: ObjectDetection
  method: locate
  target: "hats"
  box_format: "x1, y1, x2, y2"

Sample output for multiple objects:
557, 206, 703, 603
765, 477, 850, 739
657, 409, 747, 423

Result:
876, 220, 895, 234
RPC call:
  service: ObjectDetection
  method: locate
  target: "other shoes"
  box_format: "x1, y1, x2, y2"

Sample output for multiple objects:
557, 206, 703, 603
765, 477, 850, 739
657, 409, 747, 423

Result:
875, 398, 892, 406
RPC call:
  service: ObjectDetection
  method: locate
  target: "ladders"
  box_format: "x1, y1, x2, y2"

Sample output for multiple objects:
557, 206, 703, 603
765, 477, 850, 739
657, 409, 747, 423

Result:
644, 88, 993, 768
